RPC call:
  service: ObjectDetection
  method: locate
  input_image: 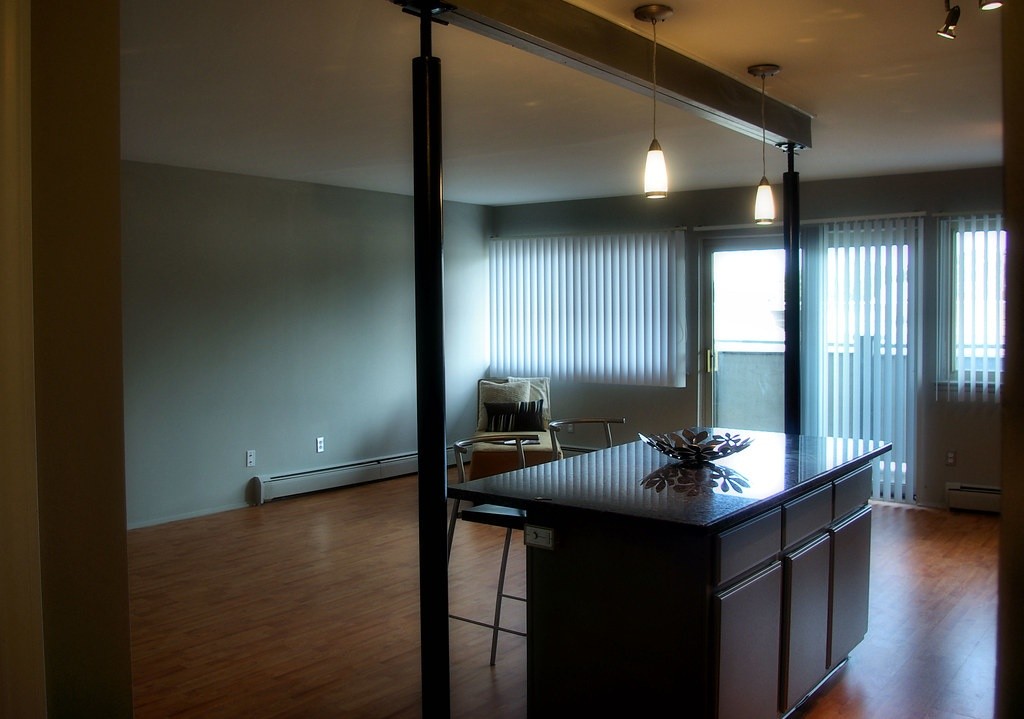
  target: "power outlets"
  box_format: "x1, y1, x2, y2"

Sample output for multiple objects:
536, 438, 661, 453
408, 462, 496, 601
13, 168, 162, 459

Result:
317, 437, 324, 452
247, 450, 256, 467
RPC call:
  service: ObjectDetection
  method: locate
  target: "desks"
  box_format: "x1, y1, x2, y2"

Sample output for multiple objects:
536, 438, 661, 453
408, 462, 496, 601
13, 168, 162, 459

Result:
447, 427, 894, 719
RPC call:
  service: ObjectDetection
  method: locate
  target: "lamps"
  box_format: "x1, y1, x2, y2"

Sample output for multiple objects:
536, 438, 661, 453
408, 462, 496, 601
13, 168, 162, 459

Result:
644, 17, 669, 199
937, 6, 961, 39
754, 74, 776, 225
979, 0, 1006, 11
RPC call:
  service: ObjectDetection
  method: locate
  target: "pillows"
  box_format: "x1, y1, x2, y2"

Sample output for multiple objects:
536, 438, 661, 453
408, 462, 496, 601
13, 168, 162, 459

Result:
478, 380, 529, 430
483, 399, 547, 431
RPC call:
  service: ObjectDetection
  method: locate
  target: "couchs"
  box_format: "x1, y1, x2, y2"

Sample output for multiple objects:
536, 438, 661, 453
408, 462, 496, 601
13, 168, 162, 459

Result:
470, 377, 564, 478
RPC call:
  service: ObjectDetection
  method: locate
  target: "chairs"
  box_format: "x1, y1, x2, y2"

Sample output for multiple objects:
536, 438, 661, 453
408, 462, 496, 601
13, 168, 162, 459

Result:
448, 434, 540, 666
549, 418, 625, 459
